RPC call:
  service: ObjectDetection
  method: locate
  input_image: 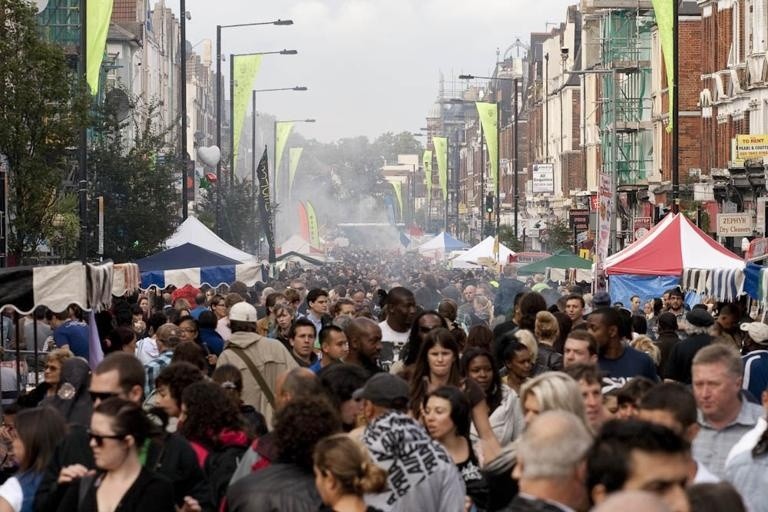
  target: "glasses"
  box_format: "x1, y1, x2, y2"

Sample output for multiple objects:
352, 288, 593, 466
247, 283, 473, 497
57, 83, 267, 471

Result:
89, 392, 125, 402
86, 433, 122, 445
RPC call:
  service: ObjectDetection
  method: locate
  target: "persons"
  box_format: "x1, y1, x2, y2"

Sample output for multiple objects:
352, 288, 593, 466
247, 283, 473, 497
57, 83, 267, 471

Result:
0, 240, 767, 512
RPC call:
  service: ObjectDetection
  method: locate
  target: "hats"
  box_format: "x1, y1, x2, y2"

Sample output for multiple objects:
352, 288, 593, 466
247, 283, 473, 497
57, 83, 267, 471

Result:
590, 292, 611, 305
740, 322, 768, 345
686, 309, 715, 327
157, 324, 180, 341
660, 313, 678, 330
229, 302, 257, 322
353, 372, 409, 407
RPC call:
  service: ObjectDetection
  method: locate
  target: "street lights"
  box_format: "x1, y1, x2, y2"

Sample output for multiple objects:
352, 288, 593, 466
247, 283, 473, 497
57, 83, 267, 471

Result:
393, 113, 484, 250
458, 74, 520, 242
229, 49, 300, 195
216, 19, 296, 250
251, 86, 310, 232
271, 116, 316, 244
436, 95, 502, 239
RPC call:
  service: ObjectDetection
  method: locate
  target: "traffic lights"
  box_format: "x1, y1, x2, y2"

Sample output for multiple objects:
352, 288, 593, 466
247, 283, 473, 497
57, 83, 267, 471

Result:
486, 196, 493, 212
